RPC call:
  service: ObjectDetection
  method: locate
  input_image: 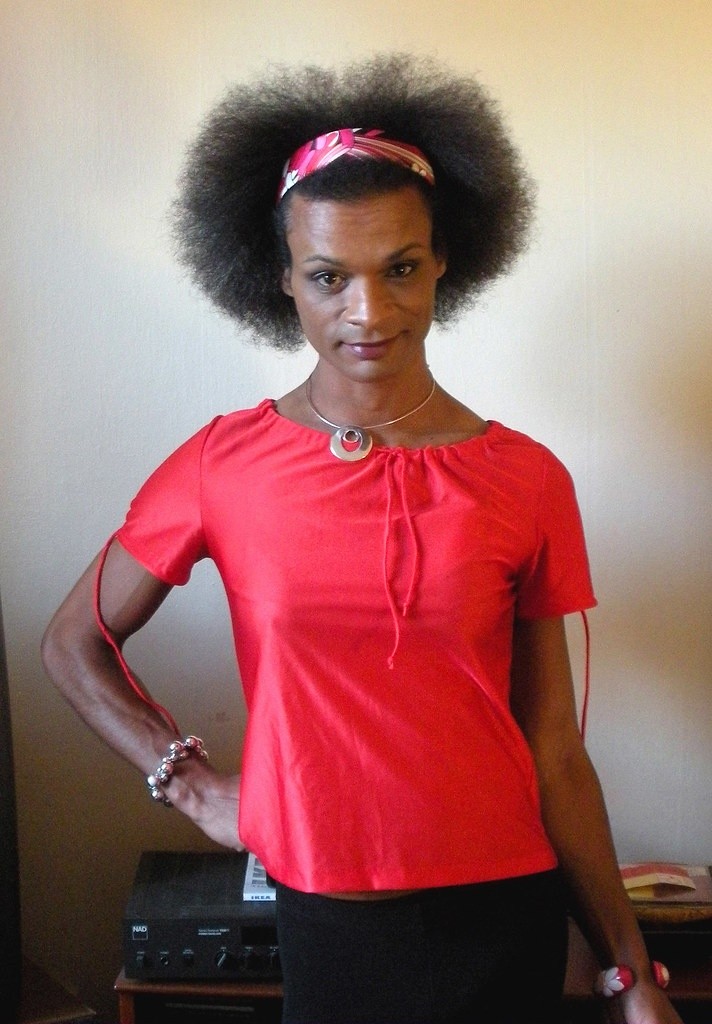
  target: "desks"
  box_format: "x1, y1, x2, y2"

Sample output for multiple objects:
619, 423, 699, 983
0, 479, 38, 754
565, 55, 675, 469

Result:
113, 909, 710, 1023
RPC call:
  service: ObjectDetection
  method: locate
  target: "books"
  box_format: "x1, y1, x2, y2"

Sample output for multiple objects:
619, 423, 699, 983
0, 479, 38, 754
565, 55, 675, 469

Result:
240, 849, 277, 902
618, 862, 712, 904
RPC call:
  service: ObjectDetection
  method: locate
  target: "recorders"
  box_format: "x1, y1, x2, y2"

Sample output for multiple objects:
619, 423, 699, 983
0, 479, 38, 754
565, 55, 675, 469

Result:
124, 844, 288, 983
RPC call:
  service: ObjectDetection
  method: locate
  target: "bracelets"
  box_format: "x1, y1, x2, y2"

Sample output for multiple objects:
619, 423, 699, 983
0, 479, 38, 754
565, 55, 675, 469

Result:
145, 732, 211, 808
592, 959, 671, 1002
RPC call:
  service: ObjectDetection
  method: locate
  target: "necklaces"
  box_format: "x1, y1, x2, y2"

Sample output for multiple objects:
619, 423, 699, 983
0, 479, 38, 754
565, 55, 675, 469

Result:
303, 375, 436, 463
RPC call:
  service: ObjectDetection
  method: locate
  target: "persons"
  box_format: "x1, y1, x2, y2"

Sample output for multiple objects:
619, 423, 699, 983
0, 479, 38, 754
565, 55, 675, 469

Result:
31, 57, 686, 1023
